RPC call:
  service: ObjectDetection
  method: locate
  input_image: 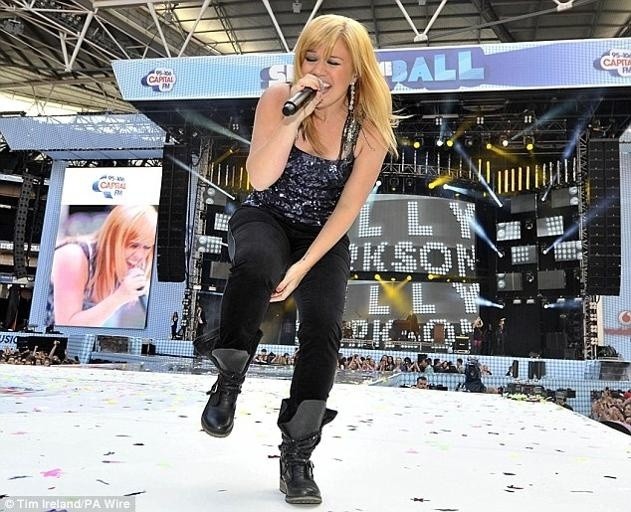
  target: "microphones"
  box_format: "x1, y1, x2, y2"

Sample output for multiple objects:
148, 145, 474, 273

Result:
281, 78, 324, 116
127, 267, 147, 313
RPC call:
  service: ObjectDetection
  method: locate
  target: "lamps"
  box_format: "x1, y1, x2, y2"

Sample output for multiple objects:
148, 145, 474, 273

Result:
195, 234, 223, 255
197, 182, 216, 205
402, 105, 536, 151
540, 242, 549, 255
229, 140, 240, 154
524, 270, 535, 283
292, 0, 303, 14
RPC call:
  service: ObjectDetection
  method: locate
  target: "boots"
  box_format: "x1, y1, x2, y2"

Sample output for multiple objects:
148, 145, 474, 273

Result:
193, 324, 263, 438
278, 399, 337, 503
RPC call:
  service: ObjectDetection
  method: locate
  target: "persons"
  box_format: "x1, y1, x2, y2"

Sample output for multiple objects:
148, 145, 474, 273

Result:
50, 204, 158, 328
200, 14, 400, 505
0, 340, 80, 367
168, 306, 631, 428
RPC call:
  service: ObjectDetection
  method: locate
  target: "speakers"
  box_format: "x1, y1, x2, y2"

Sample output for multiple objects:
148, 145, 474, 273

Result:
12, 336, 68, 358
453, 336, 470, 354
12, 173, 33, 278
94, 334, 128, 354
587, 135, 620, 296
